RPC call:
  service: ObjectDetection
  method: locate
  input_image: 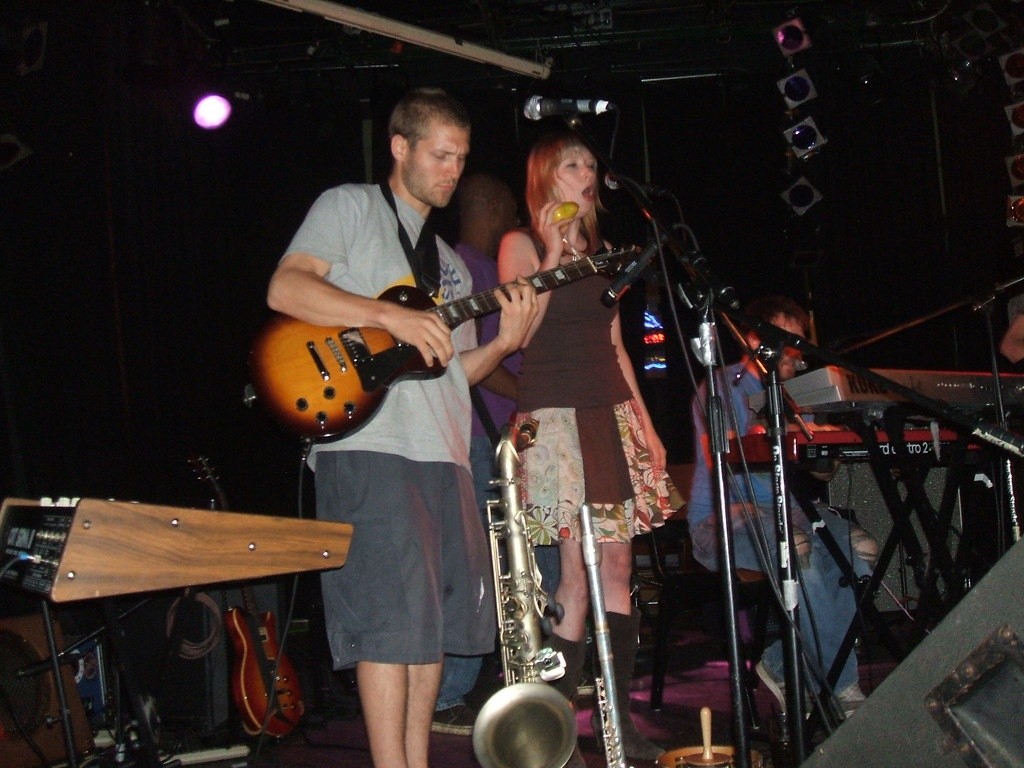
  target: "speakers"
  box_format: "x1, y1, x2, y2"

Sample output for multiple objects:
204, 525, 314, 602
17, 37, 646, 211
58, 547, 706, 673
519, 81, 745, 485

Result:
824, 442, 962, 616
0, 596, 96, 768
800, 534, 1024, 767
99, 574, 279, 737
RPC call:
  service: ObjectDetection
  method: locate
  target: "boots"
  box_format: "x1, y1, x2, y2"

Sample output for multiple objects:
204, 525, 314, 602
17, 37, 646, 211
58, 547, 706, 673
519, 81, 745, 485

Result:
543, 627, 593, 747
592, 612, 671, 761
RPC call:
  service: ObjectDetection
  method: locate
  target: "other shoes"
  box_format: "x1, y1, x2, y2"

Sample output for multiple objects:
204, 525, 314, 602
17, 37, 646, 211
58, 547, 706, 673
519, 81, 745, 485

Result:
432, 705, 476, 737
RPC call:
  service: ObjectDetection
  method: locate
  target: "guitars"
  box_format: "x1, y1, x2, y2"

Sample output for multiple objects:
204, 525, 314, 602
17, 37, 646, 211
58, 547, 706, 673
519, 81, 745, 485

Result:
186, 453, 306, 742
250, 243, 644, 445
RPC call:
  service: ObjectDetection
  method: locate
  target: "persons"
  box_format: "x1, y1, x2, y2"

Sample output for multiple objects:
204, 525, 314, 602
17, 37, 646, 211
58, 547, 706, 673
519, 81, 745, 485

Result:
266, 89, 538, 768
499, 139, 687, 768
427, 167, 520, 739
686, 302, 875, 721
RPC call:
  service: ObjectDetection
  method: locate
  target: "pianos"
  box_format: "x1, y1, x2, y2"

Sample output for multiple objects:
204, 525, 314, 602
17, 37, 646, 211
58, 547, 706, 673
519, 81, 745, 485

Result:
723, 425, 1024, 468
747, 365, 1024, 415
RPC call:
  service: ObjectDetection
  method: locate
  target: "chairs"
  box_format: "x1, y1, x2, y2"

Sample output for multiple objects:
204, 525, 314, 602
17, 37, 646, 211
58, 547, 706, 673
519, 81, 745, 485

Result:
651, 440, 775, 729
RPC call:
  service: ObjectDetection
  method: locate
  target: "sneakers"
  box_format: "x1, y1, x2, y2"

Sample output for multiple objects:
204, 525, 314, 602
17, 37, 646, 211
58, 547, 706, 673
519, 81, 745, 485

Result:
839, 681, 868, 715
754, 660, 815, 737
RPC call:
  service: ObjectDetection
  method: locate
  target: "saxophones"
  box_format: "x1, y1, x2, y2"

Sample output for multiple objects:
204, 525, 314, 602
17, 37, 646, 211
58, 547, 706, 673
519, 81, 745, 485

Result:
471, 412, 580, 768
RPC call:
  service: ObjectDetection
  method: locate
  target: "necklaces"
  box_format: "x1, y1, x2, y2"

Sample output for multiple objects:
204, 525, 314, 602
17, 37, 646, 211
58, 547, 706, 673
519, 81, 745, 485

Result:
562, 237, 582, 263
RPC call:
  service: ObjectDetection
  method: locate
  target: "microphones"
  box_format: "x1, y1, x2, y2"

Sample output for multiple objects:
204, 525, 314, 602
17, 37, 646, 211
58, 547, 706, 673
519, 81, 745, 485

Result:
523, 96, 614, 121
794, 356, 822, 372
605, 174, 674, 200
601, 226, 677, 308
